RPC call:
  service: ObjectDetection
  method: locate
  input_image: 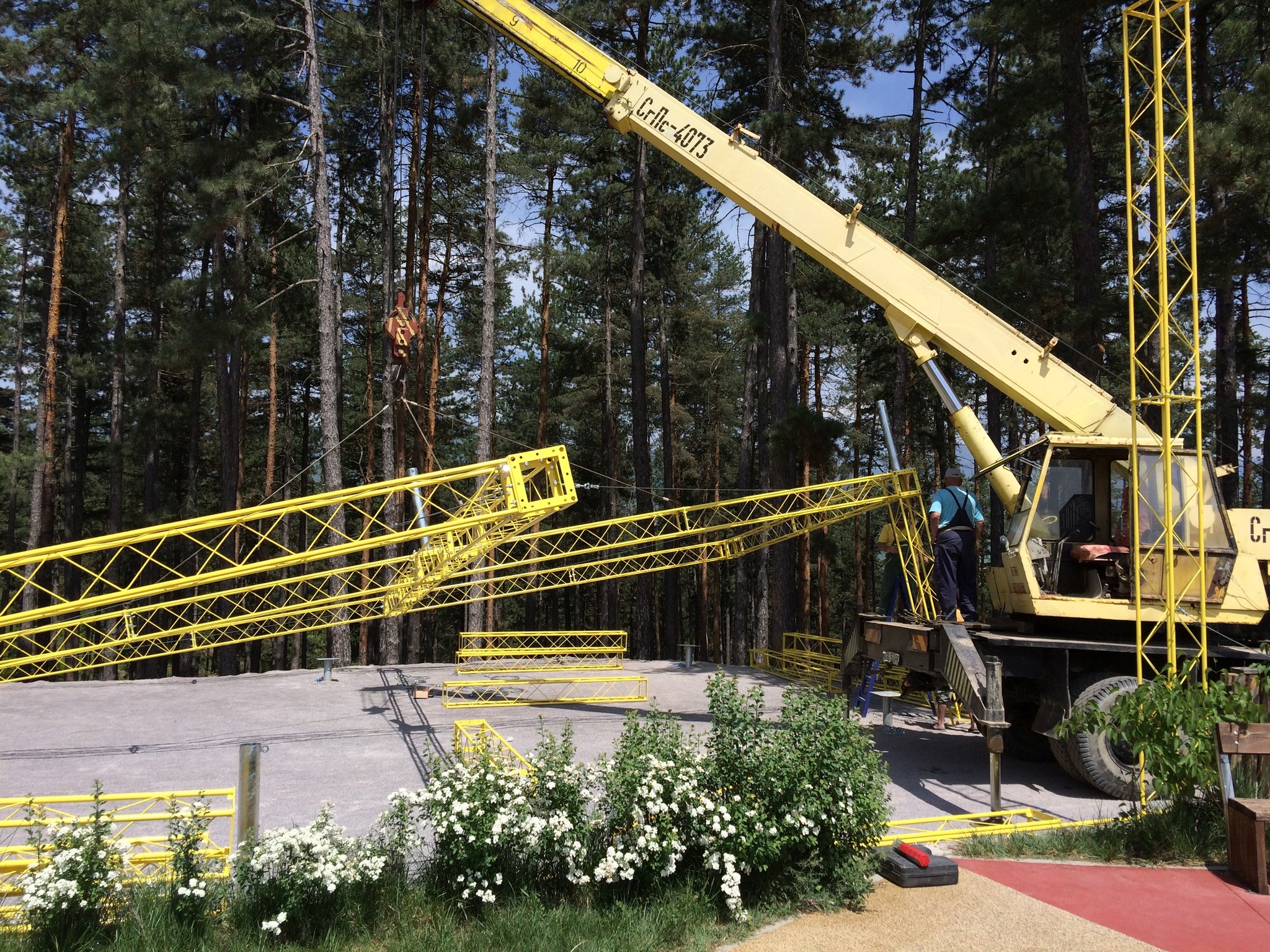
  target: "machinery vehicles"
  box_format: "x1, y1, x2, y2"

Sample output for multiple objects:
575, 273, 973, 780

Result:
383, 0, 1270, 802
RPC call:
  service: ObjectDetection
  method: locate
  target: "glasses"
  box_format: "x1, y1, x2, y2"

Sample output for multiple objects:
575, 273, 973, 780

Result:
897, 518, 903, 521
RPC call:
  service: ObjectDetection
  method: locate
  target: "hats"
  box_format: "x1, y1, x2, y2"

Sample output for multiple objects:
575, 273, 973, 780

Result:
896, 509, 908, 520
944, 468, 961, 478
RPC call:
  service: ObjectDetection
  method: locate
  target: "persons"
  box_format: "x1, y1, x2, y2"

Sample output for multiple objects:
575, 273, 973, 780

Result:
877, 509, 928, 625
904, 670, 980, 733
929, 468, 984, 621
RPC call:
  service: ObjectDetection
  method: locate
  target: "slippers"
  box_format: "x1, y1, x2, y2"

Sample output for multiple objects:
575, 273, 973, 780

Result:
929, 724, 947, 731
968, 728, 979, 733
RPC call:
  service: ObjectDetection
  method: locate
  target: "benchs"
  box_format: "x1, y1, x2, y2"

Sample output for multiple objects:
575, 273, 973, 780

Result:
1212, 722, 1270, 895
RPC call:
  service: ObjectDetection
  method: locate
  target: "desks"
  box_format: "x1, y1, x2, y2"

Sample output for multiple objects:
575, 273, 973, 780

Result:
316, 657, 339, 681
676, 645, 701, 669
871, 689, 901, 727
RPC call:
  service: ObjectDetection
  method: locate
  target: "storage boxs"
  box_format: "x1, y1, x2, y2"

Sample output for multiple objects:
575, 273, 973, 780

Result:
413, 683, 434, 698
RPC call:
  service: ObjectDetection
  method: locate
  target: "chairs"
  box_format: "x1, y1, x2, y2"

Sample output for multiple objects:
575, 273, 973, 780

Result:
1075, 486, 1153, 562
